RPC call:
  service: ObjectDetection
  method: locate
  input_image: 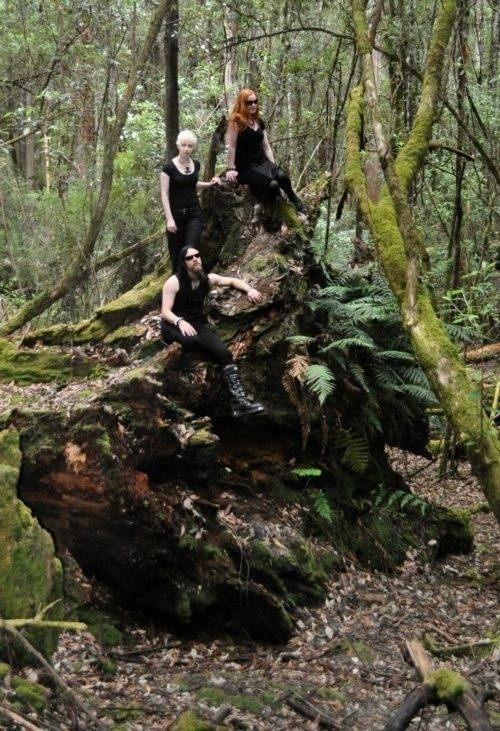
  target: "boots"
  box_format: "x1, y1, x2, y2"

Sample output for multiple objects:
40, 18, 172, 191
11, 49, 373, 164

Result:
221, 361, 267, 419
288, 190, 304, 210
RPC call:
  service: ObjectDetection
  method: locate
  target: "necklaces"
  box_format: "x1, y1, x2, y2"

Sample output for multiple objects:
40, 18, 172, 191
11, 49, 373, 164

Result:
247, 118, 258, 128
181, 160, 193, 174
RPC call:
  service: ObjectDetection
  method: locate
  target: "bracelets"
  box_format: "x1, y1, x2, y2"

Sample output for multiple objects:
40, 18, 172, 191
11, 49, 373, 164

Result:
174, 316, 186, 326
226, 167, 237, 172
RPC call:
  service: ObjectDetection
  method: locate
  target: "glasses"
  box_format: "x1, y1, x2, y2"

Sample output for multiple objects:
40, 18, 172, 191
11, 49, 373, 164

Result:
245, 97, 259, 106
184, 252, 201, 261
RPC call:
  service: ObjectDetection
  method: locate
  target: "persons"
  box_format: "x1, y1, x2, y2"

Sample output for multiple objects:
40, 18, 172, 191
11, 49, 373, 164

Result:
159, 128, 222, 274
224, 88, 303, 225
162, 244, 269, 419
